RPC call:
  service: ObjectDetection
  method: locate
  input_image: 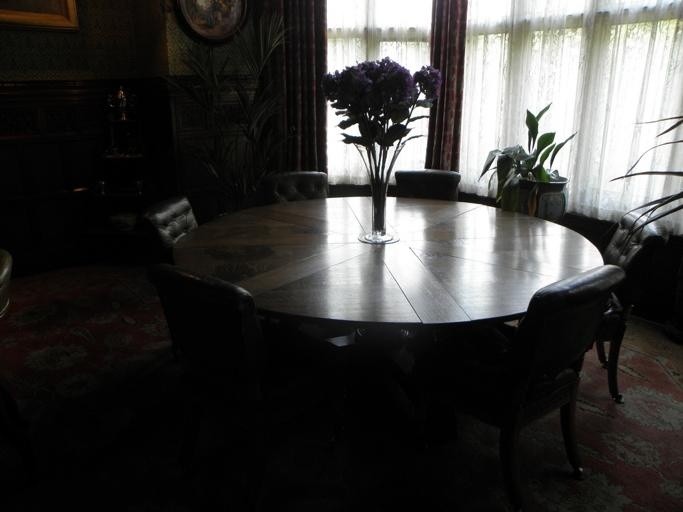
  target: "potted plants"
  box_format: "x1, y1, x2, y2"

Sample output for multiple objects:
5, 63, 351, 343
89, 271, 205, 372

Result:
477, 105, 572, 228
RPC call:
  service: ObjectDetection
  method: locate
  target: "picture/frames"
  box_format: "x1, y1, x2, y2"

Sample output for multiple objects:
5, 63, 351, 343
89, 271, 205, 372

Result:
0, 1, 79, 34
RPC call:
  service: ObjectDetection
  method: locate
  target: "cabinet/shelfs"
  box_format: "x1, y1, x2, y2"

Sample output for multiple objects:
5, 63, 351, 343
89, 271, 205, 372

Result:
0, 78, 170, 273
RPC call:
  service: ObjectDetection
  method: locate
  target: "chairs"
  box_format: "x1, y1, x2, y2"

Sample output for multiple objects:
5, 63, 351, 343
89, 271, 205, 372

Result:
163, 267, 275, 451
271, 169, 328, 200
456, 264, 623, 485
568, 205, 669, 407
396, 168, 463, 201
146, 194, 201, 286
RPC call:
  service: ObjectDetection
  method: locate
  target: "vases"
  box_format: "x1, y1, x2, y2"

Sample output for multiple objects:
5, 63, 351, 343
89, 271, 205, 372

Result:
354, 164, 400, 252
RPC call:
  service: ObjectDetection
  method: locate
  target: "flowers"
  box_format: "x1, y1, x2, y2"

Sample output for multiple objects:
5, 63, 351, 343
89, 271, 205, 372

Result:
320, 60, 449, 156
